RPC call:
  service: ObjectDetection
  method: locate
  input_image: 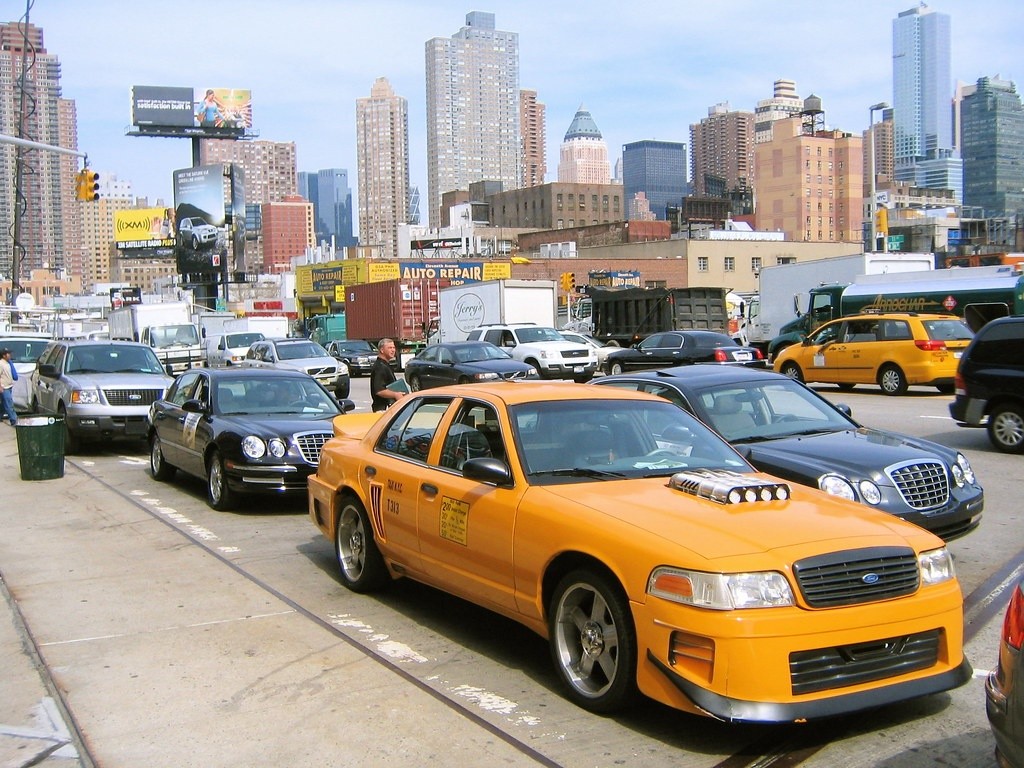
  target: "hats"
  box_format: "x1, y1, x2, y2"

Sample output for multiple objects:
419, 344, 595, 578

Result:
1, 348, 13, 355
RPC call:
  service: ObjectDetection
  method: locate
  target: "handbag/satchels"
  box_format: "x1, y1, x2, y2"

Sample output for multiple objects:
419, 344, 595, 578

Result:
386, 378, 409, 406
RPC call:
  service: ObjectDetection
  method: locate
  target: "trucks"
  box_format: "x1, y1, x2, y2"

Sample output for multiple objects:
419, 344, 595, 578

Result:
561, 285, 734, 350
421, 277, 558, 361
304, 275, 453, 372
732, 250, 937, 359
767, 251, 1024, 364
0, 300, 291, 415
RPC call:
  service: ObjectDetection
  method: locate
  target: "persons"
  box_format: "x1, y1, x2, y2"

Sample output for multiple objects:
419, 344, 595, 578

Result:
152, 209, 176, 240
261, 383, 300, 406
817, 339, 837, 354
196, 90, 245, 127
506, 333, 512, 340
370, 339, 405, 413
0, 348, 18, 425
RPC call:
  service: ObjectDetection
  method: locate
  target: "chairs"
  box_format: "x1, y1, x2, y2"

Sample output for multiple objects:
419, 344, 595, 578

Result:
74, 353, 95, 368
245, 385, 276, 409
710, 393, 756, 434
648, 390, 689, 434
217, 388, 241, 411
469, 348, 481, 360
565, 428, 623, 464
480, 427, 553, 474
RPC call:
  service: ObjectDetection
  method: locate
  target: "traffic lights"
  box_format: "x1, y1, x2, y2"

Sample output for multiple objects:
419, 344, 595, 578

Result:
74, 174, 85, 202
85, 170, 101, 202
566, 272, 576, 289
558, 295, 567, 306
560, 272, 567, 290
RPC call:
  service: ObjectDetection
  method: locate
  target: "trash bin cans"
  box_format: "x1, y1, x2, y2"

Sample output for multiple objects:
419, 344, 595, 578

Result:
14, 412, 68, 482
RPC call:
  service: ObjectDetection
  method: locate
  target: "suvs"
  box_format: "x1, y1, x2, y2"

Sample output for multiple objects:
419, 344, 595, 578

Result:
179, 216, 218, 251
243, 337, 351, 399
947, 317, 1024, 455
30, 340, 176, 456
464, 322, 599, 384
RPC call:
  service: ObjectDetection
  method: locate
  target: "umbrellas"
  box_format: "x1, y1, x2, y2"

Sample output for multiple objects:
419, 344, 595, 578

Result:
726, 294, 745, 305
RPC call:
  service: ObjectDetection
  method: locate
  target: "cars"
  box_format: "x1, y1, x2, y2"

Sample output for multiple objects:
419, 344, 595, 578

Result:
602, 329, 767, 377
405, 340, 540, 393
145, 366, 356, 511
524, 363, 985, 544
321, 340, 398, 378
771, 312, 976, 397
305, 380, 975, 726
547, 332, 630, 372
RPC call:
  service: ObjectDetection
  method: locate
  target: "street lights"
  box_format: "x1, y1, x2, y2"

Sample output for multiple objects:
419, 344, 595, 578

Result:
867, 101, 891, 253
494, 224, 503, 257
